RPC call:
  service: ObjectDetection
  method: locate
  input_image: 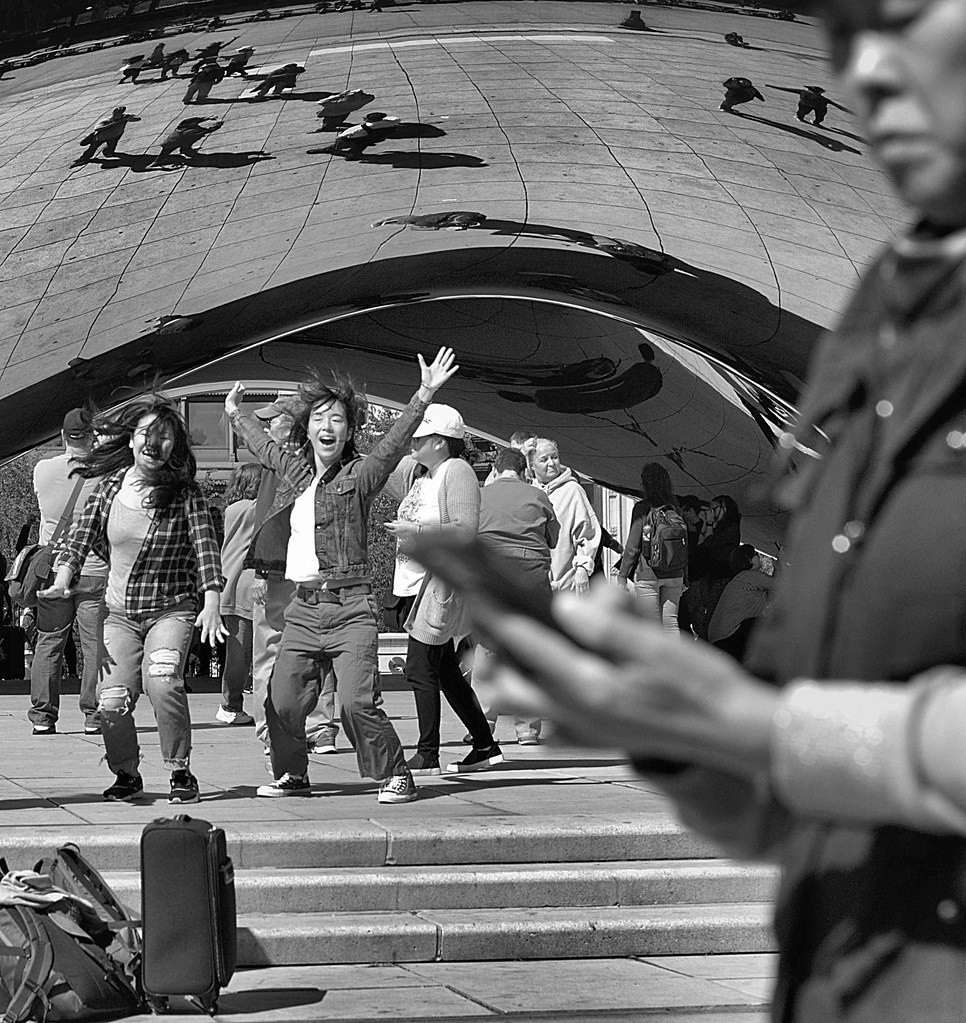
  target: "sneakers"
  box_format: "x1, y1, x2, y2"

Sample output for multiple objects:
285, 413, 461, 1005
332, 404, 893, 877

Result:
103, 768, 144, 801
216, 702, 254, 725
378, 773, 417, 803
404, 753, 441, 776
256, 772, 311, 797
307, 736, 338, 753
167, 770, 200, 803
446, 739, 504, 772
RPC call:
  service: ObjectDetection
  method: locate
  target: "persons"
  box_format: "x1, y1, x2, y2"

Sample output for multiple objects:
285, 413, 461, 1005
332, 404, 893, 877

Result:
725, 31, 749, 48
577, 234, 675, 277
192, 506, 226, 681
377, 404, 505, 776
765, 83, 854, 127
370, 211, 486, 232
119, 0, 384, 103
68, 106, 142, 169
460, 447, 561, 746
224, 346, 460, 802
36, 394, 229, 804
718, 77, 765, 112
306, 89, 375, 134
142, 114, 224, 170
307, 112, 401, 160
452, 0, 966, 1023
589, 461, 774, 663
26, 408, 106, 736
251, 396, 341, 776
518, 437, 601, 597
215, 463, 266, 725
484, 430, 536, 486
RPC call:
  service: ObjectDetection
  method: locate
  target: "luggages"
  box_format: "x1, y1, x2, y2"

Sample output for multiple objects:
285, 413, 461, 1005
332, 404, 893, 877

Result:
0, 599, 25, 681
141, 815, 237, 1018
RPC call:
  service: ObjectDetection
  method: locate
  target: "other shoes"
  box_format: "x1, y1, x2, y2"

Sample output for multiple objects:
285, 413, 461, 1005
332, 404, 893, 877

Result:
463, 733, 474, 744
85, 726, 103, 734
518, 735, 543, 745
32, 723, 55, 735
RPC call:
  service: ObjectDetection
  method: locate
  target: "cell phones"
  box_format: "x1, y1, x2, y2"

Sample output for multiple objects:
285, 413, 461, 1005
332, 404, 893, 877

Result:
404, 527, 572, 683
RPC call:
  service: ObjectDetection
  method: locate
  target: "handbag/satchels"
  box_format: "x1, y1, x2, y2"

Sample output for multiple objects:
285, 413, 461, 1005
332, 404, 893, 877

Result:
3, 543, 52, 608
383, 591, 416, 633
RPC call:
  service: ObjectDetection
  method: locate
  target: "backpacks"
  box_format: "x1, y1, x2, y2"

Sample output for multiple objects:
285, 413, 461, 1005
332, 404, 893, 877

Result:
35, 838, 143, 993
643, 499, 688, 571
0, 858, 140, 1023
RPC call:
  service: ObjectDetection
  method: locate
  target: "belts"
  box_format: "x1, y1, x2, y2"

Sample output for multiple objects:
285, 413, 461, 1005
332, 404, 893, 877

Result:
295, 587, 372, 605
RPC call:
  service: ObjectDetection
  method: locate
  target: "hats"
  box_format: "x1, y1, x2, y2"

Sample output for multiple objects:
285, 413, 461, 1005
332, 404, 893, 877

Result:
253, 398, 308, 422
408, 403, 467, 439
63, 408, 93, 439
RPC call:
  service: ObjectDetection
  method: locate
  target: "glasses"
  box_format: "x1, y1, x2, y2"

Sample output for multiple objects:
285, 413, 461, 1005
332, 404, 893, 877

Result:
751, 552, 759, 560
712, 505, 724, 513
412, 435, 435, 443
266, 419, 278, 425
